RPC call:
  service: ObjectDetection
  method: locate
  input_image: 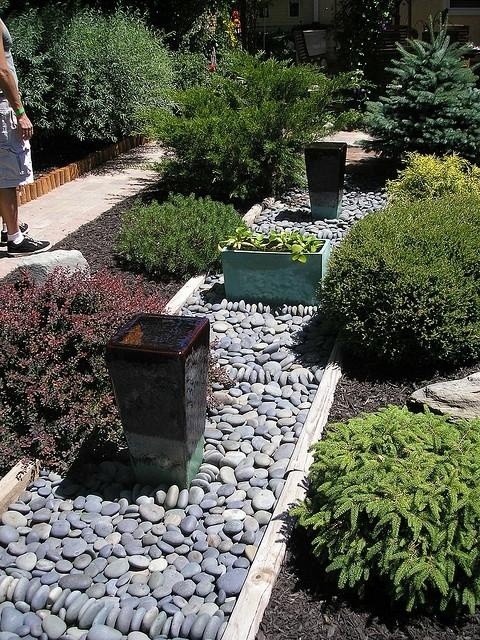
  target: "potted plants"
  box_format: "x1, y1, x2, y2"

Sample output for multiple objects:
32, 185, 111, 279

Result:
219, 225, 330, 306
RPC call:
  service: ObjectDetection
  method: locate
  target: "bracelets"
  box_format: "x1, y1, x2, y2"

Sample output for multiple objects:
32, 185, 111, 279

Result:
14, 106, 25, 117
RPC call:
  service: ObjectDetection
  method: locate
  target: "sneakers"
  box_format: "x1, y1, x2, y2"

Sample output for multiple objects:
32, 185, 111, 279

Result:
1, 221, 30, 246
7, 234, 51, 258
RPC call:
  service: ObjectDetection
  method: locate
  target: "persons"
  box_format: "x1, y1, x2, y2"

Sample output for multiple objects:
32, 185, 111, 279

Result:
0, 17, 54, 258
